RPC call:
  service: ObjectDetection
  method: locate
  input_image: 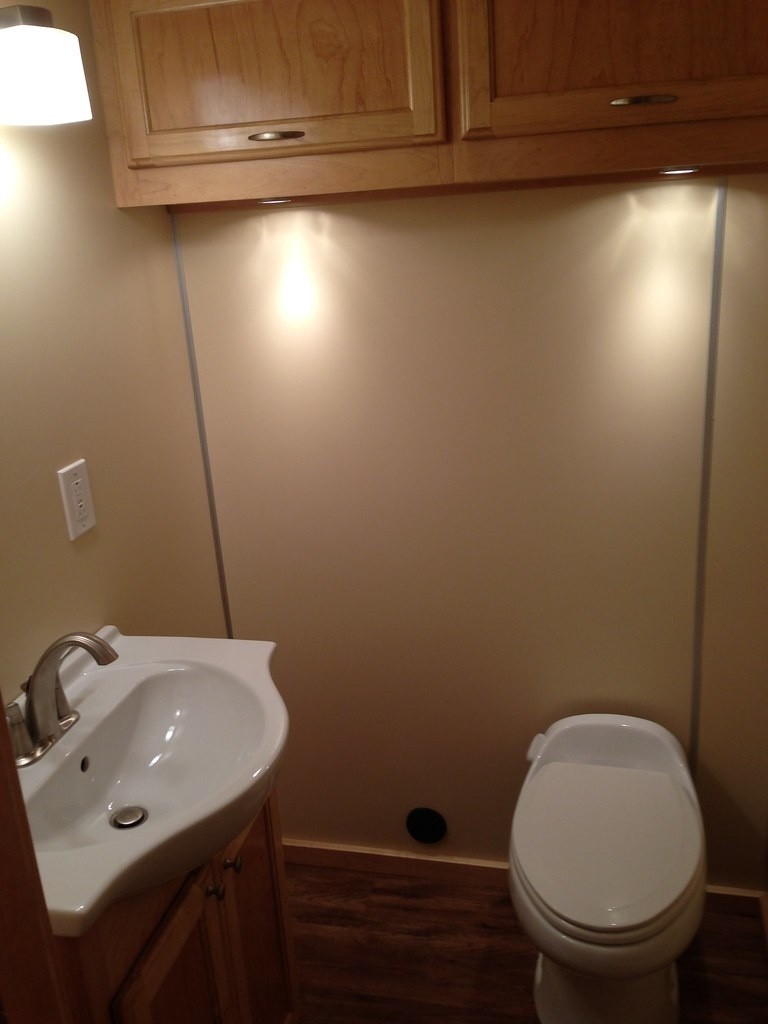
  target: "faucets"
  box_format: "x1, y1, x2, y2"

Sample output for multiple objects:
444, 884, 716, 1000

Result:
26, 630, 119, 741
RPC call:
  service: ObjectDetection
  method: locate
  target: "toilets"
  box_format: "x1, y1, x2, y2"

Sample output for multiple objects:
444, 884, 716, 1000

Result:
507, 711, 706, 1024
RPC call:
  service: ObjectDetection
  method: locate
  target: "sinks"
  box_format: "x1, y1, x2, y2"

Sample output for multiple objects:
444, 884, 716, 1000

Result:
25, 626, 291, 941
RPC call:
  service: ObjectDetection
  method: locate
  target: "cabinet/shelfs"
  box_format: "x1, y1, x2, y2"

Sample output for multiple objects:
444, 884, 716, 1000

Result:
87, 1, 768, 209
108, 786, 301, 1023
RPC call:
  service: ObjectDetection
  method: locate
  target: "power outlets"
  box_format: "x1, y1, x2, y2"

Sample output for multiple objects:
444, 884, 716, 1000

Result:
58, 456, 98, 540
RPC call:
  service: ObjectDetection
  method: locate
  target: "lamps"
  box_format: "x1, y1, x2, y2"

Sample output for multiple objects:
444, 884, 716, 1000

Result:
0, 3, 94, 128
